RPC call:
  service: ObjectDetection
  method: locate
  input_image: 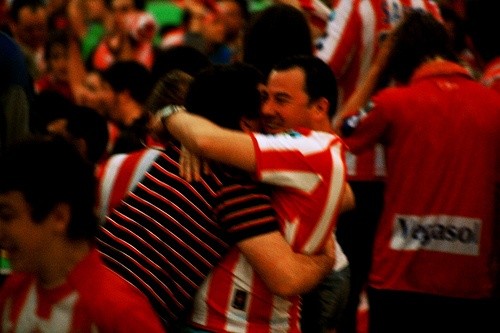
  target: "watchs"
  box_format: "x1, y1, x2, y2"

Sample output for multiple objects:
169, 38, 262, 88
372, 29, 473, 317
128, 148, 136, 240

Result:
161, 103, 183, 134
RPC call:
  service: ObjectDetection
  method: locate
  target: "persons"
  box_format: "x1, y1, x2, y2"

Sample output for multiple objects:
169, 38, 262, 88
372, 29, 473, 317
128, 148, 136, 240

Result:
0, 0, 500, 333
